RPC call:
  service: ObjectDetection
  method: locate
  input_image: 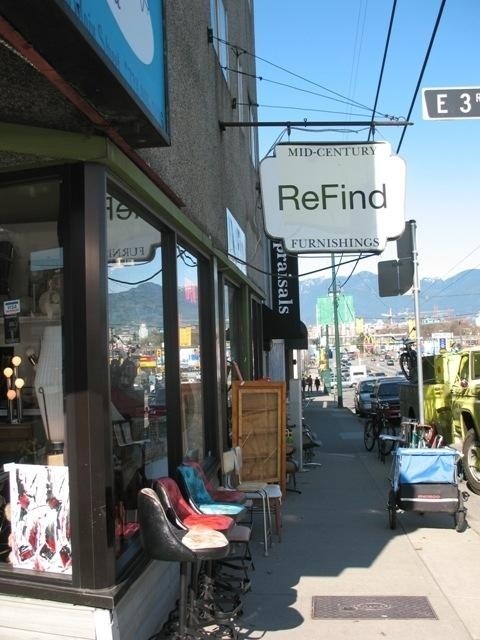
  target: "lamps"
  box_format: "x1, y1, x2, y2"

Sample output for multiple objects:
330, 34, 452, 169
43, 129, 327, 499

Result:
2, 355, 25, 425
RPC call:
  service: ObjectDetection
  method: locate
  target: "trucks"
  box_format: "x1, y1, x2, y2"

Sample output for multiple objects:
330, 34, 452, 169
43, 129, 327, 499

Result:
399, 344, 480, 495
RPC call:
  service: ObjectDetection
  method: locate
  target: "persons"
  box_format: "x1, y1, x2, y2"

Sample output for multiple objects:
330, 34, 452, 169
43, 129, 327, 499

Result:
302, 375, 320, 392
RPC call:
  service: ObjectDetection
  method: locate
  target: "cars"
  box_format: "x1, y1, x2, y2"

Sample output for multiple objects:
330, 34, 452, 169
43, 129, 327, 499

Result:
145, 384, 166, 432
309, 339, 404, 419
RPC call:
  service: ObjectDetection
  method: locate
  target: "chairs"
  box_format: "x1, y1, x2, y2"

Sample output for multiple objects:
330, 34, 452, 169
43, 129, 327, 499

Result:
376, 416, 415, 464
131, 446, 283, 640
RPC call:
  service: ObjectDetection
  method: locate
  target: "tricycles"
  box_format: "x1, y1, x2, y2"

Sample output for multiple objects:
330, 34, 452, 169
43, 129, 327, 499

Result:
389, 422, 469, 532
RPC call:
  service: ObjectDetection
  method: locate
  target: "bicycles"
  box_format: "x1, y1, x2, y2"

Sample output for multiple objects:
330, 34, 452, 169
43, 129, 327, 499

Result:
363, 399, 395, 456
